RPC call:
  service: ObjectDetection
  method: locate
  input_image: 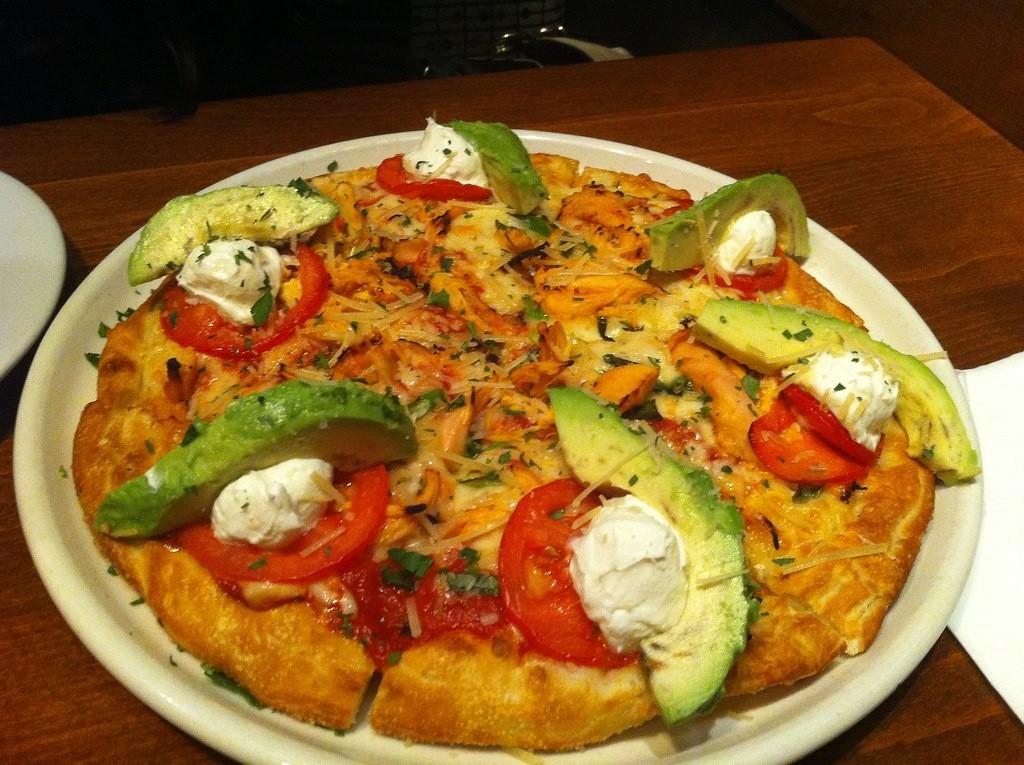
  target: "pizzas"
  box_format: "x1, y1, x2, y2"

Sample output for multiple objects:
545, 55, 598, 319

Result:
71, 116, 980, 752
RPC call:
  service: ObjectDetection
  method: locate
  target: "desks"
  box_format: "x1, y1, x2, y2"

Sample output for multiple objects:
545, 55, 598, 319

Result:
0, 32, 1024, 765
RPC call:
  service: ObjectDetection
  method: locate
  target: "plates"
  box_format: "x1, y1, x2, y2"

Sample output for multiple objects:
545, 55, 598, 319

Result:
13, 129, 983, 765
0, 171, 67, 381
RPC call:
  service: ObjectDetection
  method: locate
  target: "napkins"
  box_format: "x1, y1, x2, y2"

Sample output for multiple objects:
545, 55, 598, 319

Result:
945, 349, 1024, 724
